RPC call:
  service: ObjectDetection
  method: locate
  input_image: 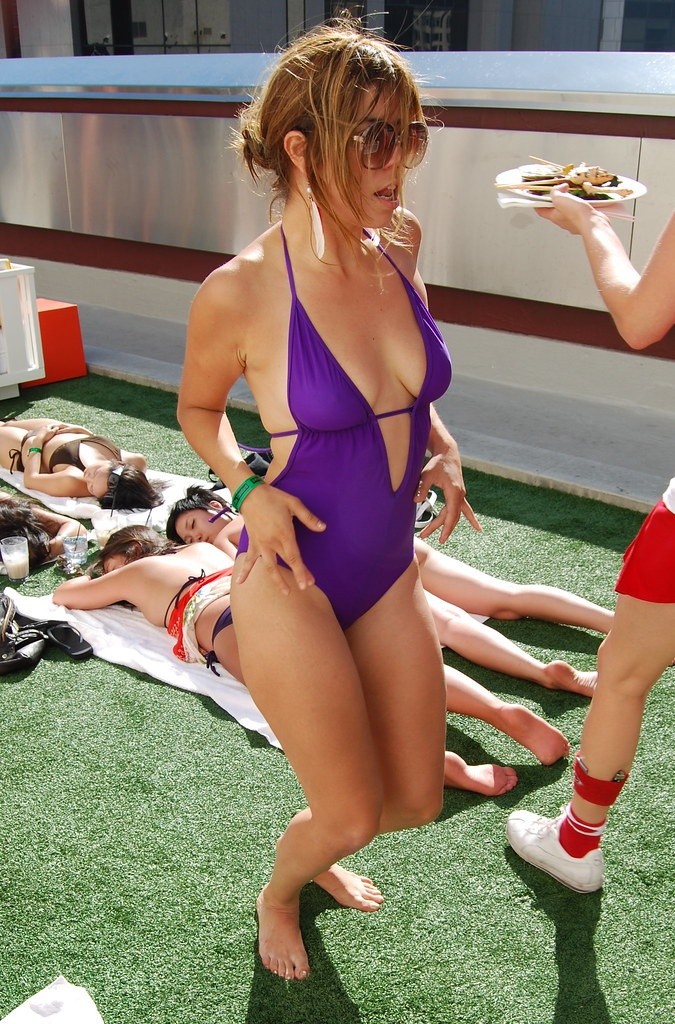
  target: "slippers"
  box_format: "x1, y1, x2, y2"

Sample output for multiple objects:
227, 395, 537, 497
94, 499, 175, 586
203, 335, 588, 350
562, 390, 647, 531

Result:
47, 621, 93, 660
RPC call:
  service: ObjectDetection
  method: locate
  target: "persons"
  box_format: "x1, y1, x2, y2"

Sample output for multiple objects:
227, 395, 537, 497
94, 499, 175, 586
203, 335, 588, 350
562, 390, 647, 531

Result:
0, 491, 87, 566
177, 28, 483, 981
506, 183, 675, 894
166, 486, 675, 697
0, 419, 166, 510
53, 525, 570, 795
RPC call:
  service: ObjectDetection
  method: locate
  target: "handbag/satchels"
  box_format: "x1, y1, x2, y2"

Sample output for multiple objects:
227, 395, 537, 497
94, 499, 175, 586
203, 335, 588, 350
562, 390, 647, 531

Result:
0, 593, 49, 677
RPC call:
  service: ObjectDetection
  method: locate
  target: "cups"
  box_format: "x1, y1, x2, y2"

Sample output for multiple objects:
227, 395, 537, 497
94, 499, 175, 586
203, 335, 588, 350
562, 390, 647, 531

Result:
126, 514, 152, 527
91, 510, 122, 548
63, 531, 88, 564
0, 536, 29, 582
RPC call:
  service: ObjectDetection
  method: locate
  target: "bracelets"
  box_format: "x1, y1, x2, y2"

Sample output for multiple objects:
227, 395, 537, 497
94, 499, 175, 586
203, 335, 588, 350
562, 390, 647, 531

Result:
232, 475, 265, 512
29, 448, 42, 453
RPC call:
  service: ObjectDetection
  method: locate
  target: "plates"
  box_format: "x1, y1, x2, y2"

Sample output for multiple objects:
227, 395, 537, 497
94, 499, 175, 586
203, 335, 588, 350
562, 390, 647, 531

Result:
494, 164, 648, 208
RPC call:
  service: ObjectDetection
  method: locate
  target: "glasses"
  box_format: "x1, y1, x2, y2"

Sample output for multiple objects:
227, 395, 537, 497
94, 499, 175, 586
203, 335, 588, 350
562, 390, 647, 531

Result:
300, 120, 429, 171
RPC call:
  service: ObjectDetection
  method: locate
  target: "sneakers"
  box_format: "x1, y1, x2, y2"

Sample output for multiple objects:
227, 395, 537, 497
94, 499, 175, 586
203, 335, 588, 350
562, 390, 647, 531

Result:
506, 809, 604, 893
